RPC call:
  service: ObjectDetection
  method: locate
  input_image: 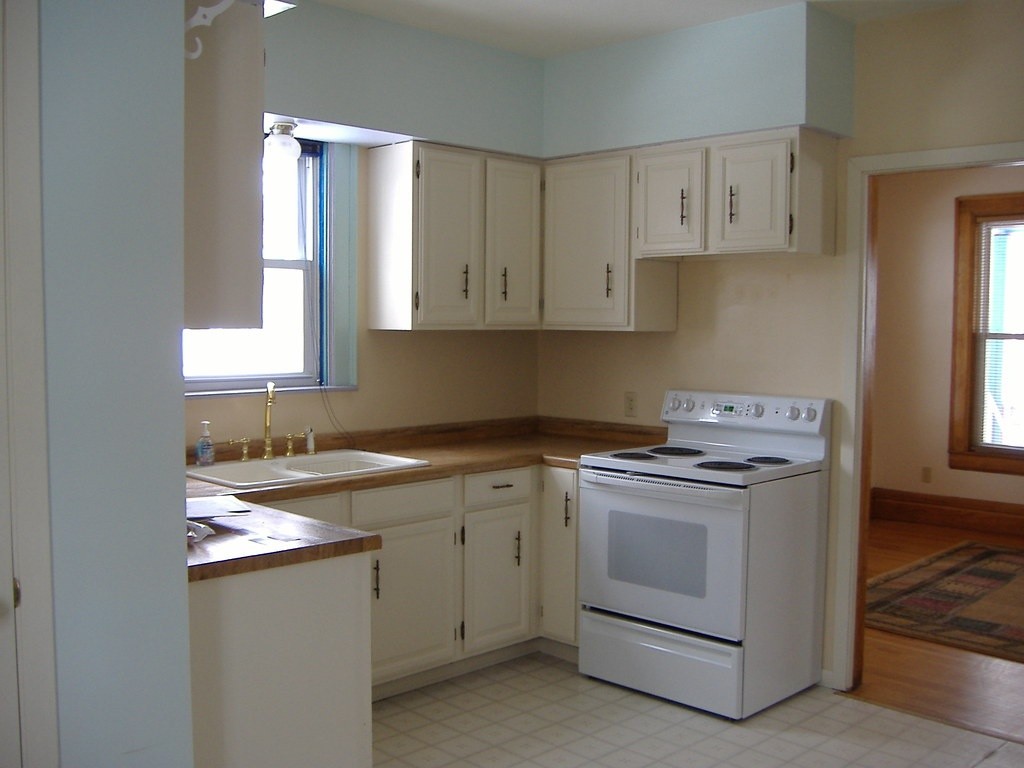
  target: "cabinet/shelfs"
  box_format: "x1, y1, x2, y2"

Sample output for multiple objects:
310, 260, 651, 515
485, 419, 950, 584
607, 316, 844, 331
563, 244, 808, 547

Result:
366, 141, 543, 331
460, 465, 539, 676
632, 126, 839, 260
185, 0, 268, 330
539, 463, 581, 666
542, 149, 679, 332
256, 475, 459, 703
188, 551, 373, 768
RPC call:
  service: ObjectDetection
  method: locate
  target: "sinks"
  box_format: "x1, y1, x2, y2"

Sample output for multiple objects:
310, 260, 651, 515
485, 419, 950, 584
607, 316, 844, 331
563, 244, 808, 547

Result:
279, 447, 431, 478
185, 458, 301, 489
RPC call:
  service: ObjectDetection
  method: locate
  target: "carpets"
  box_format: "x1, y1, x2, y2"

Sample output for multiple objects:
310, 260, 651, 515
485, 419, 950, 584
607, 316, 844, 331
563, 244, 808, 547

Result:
863, 539, 1023, 664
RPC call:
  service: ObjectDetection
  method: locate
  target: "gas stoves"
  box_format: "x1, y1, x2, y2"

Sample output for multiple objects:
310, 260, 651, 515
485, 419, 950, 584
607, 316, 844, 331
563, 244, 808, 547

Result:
579, 389, 831, 486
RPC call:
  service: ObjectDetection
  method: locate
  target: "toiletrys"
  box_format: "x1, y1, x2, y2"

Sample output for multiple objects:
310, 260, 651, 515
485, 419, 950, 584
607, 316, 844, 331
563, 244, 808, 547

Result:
195, 420, 215, 466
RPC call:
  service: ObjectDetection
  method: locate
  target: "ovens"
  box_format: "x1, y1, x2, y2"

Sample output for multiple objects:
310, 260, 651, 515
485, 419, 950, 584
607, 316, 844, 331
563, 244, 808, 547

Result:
578, 471, 830, 722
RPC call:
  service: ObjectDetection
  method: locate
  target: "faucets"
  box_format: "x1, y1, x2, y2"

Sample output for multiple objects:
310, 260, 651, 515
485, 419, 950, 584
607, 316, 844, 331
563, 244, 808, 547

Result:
261, 380, 277, 460
